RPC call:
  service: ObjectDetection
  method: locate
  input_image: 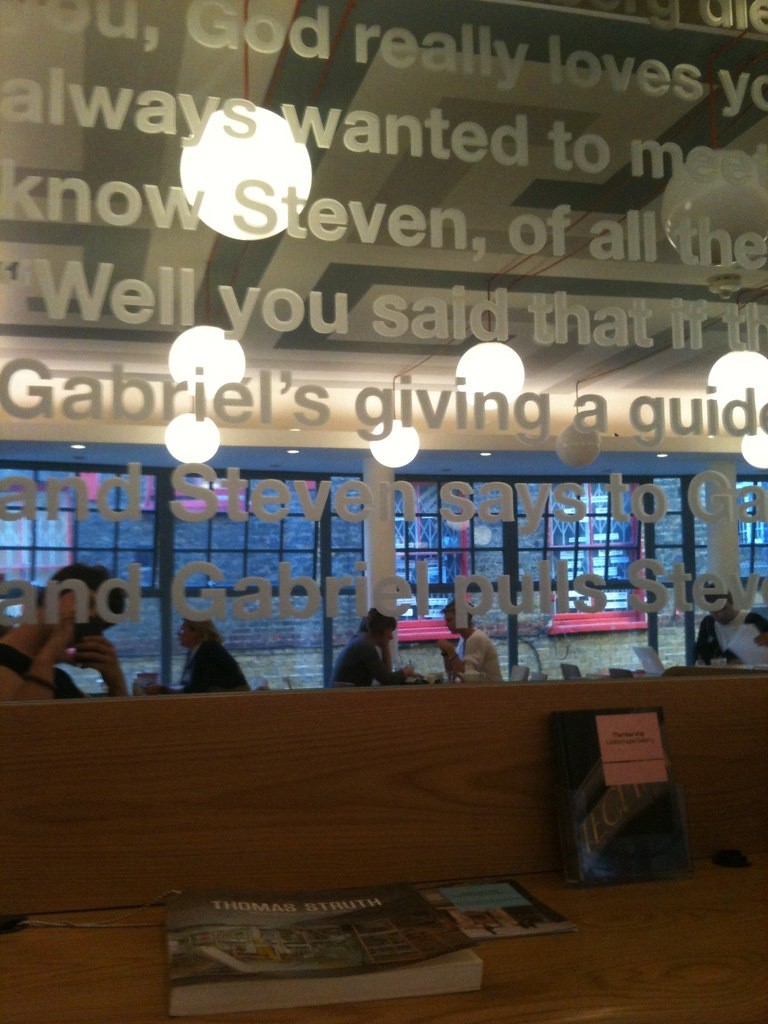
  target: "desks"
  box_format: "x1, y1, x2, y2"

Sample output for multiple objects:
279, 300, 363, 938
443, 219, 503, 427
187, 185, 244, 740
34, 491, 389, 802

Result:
0, 674, 768, 1024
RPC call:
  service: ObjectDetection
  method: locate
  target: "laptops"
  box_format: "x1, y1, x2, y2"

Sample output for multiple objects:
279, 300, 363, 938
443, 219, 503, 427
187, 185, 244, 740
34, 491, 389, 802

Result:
632, 645, 667, 674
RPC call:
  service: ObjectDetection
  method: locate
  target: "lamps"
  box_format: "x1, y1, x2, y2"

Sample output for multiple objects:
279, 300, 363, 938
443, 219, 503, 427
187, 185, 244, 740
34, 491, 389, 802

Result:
168, 263, 248, 400
707, 294, 768, 470
164, 412, 221, 464
555, 381, 602, 467
368, 375, 419, 469
456, 277, 524, 411
180, 0, 313, 241
657, 45, 768, 268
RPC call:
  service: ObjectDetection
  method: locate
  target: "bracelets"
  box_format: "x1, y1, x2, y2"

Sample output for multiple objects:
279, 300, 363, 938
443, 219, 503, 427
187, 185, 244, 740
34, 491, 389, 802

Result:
23, 673, 57, 692
445, 654, 458, 664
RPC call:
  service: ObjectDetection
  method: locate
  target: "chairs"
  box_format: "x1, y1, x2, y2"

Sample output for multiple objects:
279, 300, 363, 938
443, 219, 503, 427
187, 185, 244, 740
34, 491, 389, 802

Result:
509, 665, 548, 682
560, 663, 581, 681
608, 667, 633, 679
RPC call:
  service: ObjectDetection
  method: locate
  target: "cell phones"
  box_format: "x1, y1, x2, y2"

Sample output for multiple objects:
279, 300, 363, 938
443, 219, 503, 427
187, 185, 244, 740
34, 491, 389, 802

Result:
74, 616, 104, 670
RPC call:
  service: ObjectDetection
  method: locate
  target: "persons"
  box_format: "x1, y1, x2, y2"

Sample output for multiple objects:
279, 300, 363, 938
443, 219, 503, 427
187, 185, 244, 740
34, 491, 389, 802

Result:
142, 616, 251, 696
436, 599, 503, 683
0, 564, 130, 702
331, 608, 418, 688
692, 575, 768, 668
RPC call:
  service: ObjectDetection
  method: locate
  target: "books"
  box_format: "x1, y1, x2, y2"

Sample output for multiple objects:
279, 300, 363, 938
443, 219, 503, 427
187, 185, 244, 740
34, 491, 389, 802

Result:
164, 882, 485, 1016
549, 706, 691, 886
